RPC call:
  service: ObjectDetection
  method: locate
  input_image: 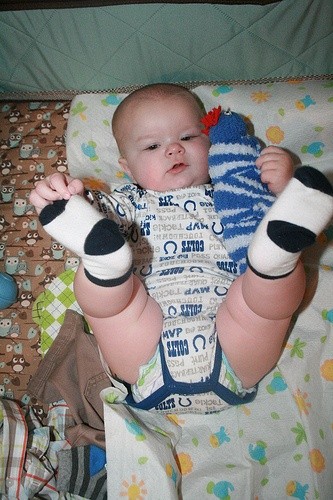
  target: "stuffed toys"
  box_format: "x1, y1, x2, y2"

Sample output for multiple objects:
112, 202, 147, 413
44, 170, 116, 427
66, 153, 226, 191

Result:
200, 103, 278, 274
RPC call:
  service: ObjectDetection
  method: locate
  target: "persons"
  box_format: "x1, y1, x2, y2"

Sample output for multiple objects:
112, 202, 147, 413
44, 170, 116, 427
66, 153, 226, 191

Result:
27, 83, 333, 415
